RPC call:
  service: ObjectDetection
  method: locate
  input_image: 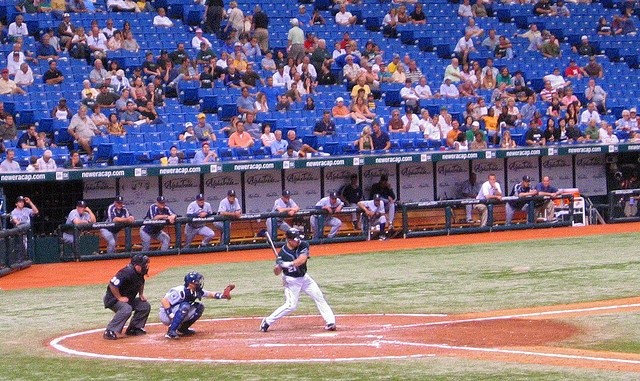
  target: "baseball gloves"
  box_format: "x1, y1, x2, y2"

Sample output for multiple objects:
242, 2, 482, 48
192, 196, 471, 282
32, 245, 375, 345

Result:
223, 285, 235, 299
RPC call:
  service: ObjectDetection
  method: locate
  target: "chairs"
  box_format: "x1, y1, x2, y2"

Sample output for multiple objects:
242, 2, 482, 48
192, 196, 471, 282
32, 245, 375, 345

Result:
449, 108, 640, 149
460, 0, 640, 68
0, 84, 289, 171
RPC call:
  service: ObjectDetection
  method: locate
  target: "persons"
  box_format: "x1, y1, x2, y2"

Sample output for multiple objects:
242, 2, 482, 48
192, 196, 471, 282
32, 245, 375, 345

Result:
371, 55, 387, 71
141, 100, 166, 125
47, 27, 61, 52
399, 77, 421, 100
233, 32, 249, 54
131, 76, 147, 99
0, 15, 11, 45
343, 55, 367, 84
189, 58, 199, 77
443, 57, 466, 83
42, 60, 65, 85
436, 105, 453, 138
107, 30, 123, 51
560, 87, 581, 107
612, 13, 637, 36
25, 156, 40, 172
597, 120, 608, 137
565, 103, 578, 122
216, 52, 235, 70
381, 7, 399, 38
348, 40, 361, 58
243, 14, 253, 31
45, 97, 70, 139
105, 113, 127, 136
377, 64, 392, 79
556, 117, 571, 141
545, 97, 561, 117
297, 0, 362, 11
401, 52, 421, 72
515, 112, 523, 119
461, 173, 481, 224
157, 49, 175, 68
36, 149, 57, 172
0, 148, 23, 173
360, 41, 378, 65
243, 35, 262, 57
169, 43, 191, 65
357, 193, 388, 241
308, 9, 327, 27
265, 189, 300, 243
270, 129, 288, 155
358, 125, 375, 151
601, 123, 619, 143
281, 145, 298, 158
316, 59, 339, 85
540, 34, 563, 59
369, 173, 397, 231
534, 0, 558, 17
465, 120, 488, 141
579, 55, 603, 79
490, 97, 503, 115
365, 74, 375, 89
101, 18, 118, 38
446, 119, 463, 148
191, 28, 213, 50
331, 95, 374, 125
214, 73, 226, 88
385, 53, 401, 74
67, 105, 105, 155
105, 0, 156, 13
222, 58, 244, 80
401, 105, 424, 133
470, 132, 488, 149
309, 191, 345, 240
231, 42, 247, 61
388, 108, 407, 132
482, 68, 496, 90
405, 60, 424, 83
159, 145, 183, 166
539, 80, 571, 102
178, 56, 202, 81
510, 69, 526, 86
218, 115, 246, 138
332, 42, 346, 59
543, 67, 572, 90
542, 29, 559, 45
16, 124, 58, 149
287, 72, 306, 95
13, 62, 35, 88
505, 174, 539, 227
106, 61, 119, 78
295, 4, 311, 27
275, 49, 288, 69
462, 101, 480, 120
0, 68, 28, 95
152, 7, 175, 28
0, 137, 6, 153
311, 38, 331, 76
58, 12, 75, 54
253, 93, 269, 112
517, 119, 528, 130
335, 3, 357, 26
139, 195, 178, 252
304, 32, 318, 52
528, 110, 543, 126
439, 76, 460, 98
353, 120, 391, 151
193, 0, 206, 12
579, 101, 602, 125
0, 101, 10, 126
396, 5, 410, 24
340, 31, 352, 49
133, 88, 148, 107
249, 76, 281, 109
223, 1, 245, 45
480, 107, 498, 130
482, 57, 500, 77
177, 121, 193, 142
81, 79, 99, 101
490, 81, 516, 102
297, 55, 318, 81
120, 100, 147, 129
520, 94, 537, 119
233, 51, 247, 75
464, 18, 484, 37
90, 104, 110, 135
424, 114, 444, 141
564, 59, 589, 81
299, 76, 318, 94
414, 77, 441, 99
99, 195, 136, 254
6, 43, 38, 73
86, 19, 103, 37
408, 2, 426, 26
531, 175, 564, 224
282, 57, 298, 75
470, 60, 483, 76
236, 87, 258, 122
196, 41, 217, 65
95, 83, 116, 108
10, 35, 31, 57
615, 109, 633, 134
352, 96, 377, 118
259, 123, 276, 147
493, 35, 514, 60
497, 105, 516, 126
98, 74, 116, 93
350, 76, 371, 100
544, 117, 557, 142
243, 111, 262, 140
212, 189, 243, 245
87, 26, 108, 51
156, 269, 236, 340
388, 64, 406, 83
71, 26, 88, 58
251, 3, 269, 53
505, 97, 519, 115
285, 82, 302, 104
257, 227, 337, 331
460, 61, 472, 79
363, 64, 380, 83
115, 88, 138, 111
192, 141, 218, 164
193, 112, 217, 141
300, 63, 314, 84
374, 43, 382, 54
102, 253, 151, 340
228, 120, 254, 150
481, 29, 513, 48
596, 14, 612, 35
289, 66, 297, 77
614, 107, 640, 131
366, 93, 378, 114
495, 66, 513, 88
453, 28, 478, 63
121, 21, 133, 40
89, 58, 108, 84
286, 17, 305, 60
261, 50, 277, 71
208, 58, 227, 79
14, 0, 97, 14
570, 35, 597, 56
453, 132, 468, 151
459, 115, 473, 131
347, 88, 367, 111
359, 54, 371, 72
89, 50, 106, 67
507, 80, 537, 104
419, 107, 432, 129
471, 0, 489, 18
301, 96, 316, 111
584, 79, 607, 115
340, 43, 362, 65
9, 195, 39, 251
183, 193, 216, 248
470, 69, 483, 90
242, 64, 267, 88
35, 33, 60, 60
340, 173, 364, 231
585, 117, 601, 142
525, 119, 545, 146
160, 60, 185, 104
128, 68, 146, 87
457, 0, 473, 17
313, 110, 336, 137
62, 200, 97, 245
275, 94, 291, 114
81, 89, 96, 111
203, 0, 226, 33
551, 0, 570, 18
63, 150, 84, 170
110, 68, 131, 95
473, 96, 489, 115
6, 14, 29, 40
141, 50, 161, 83
457, 79, 481, 97
608, 16, 624, 36
225, 64, 252, 88
608, 0, 640, 17
565, 117, 590, 142
306, 47, 315, 60
123, 30, 140, 52
199, 65, 212, 88
0, 114, 18, 140
145, 83, 166, 107
286, 129, 331, 158
513, 23, 543, 53
499, 130, 517, 148
272, 63, 291, 88
474, 173, 503, 227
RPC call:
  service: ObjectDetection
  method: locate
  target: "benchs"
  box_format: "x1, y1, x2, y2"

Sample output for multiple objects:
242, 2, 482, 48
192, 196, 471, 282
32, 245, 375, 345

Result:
251, 214, 363, 244
453, 203, 547, 230
358, 206, 472, 234
167, 220, 263, 248
81, 225, 171, 255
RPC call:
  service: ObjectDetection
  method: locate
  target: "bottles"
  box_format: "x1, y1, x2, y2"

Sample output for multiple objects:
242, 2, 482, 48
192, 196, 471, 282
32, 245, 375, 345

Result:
505, 142, 509, 149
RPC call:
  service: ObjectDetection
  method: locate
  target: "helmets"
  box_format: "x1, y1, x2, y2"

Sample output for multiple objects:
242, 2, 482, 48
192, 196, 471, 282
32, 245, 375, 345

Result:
285, 227, 302, 236
131, 252, 150, 276
184, 270, 205, 291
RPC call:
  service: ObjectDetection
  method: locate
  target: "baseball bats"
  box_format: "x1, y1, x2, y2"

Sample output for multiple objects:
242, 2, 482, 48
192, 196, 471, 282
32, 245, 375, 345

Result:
265, 231, 279, 258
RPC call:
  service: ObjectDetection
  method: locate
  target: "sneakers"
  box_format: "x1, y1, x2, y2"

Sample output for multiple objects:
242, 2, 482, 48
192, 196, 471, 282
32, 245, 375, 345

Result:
164, 330, 180, 339
260, 318, 270, 331
325, 323, 337, 330
176, 326, 196, 336
125, 325, 147, 335
104, 329, 119, 339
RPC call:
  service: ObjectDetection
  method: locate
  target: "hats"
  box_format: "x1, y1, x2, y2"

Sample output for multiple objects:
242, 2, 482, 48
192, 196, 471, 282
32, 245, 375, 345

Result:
235, 41, 242, 46
472, 120, 479, 126
76, 200, 87, 206
581, 34, 588, 39
282, 189, 291, 195
368, 92, 375, 96
202, 140, 210, 146
228, 189, 235, 195
335, 96, 344, 102
441, 105, 448, 109
523, 175, 530, 180
365, 40, 375, 47
630, 107, 637, 112
330, 191, 337, 198
198, 112, 206, 118
186, 121, 193, 128
114, 194, 124, 200
12, 52, 19, 55
570, 58, 576, 61
381, 174, 389, 181
196, 28, 202, 32
290, 17, 298, 24
515, 79, 521, 84
157, 196, 166, 202
15, 195, 25, 202
196, 192, 204, 199
373, 193, 382, 200
406, 77, 412, 82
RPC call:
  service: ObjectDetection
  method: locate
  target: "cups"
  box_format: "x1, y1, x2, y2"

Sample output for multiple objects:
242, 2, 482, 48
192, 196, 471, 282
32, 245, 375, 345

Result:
539, 140, 543, 147
439, 145, 445, 151
306, 153, 311, 158
620, 139, 625, 143
162, 157, 167, 164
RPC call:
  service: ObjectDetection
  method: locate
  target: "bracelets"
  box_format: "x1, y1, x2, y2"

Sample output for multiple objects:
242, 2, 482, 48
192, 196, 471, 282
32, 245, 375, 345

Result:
153, 112, 157, 116
72, 133, 76, 137
263, 84, 267, 88
495, 188, 498, 191
215, 292, 222, 298
99, 131, 102, 135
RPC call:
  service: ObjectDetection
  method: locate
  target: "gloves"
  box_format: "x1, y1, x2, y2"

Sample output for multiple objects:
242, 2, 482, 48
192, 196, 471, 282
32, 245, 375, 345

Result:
281, 260, 292, 268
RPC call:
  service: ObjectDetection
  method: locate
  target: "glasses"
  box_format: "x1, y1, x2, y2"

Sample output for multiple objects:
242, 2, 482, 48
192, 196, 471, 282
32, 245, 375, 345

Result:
394, 113, 400, 116
108, 23, 113, 25
